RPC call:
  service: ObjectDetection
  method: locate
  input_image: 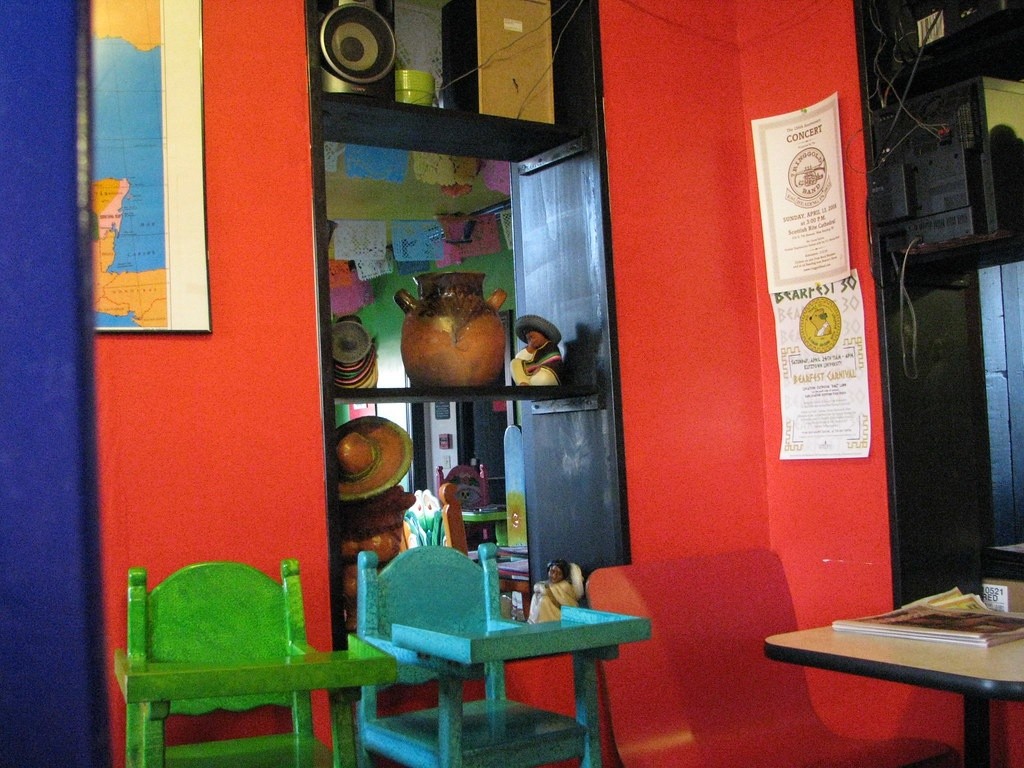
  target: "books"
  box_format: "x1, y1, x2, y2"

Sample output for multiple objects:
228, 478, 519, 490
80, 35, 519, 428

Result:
829, 586, 1024, 647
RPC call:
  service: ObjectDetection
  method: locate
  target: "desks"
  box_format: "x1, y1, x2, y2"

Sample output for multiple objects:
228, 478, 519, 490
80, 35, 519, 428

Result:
462, 512, 508, 547
765, 611, 1024, 768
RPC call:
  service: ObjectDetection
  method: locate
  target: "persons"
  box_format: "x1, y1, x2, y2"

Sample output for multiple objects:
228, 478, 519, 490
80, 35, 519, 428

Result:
524, 559, 579, 626
507, 315, 562, 385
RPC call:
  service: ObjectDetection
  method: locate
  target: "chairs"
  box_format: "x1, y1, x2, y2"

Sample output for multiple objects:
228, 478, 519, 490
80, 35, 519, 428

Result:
357, 542, 651, 768
115, 558, 396, 768
436, 464, 491, 542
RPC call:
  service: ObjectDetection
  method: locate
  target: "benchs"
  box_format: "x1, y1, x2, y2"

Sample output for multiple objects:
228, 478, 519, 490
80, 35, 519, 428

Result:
586, 548, 951, 768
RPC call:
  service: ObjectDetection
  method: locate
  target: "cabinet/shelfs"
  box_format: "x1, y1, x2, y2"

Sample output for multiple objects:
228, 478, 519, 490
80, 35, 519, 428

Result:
305, 0, 631, 702
853, 0, 1024, 611
